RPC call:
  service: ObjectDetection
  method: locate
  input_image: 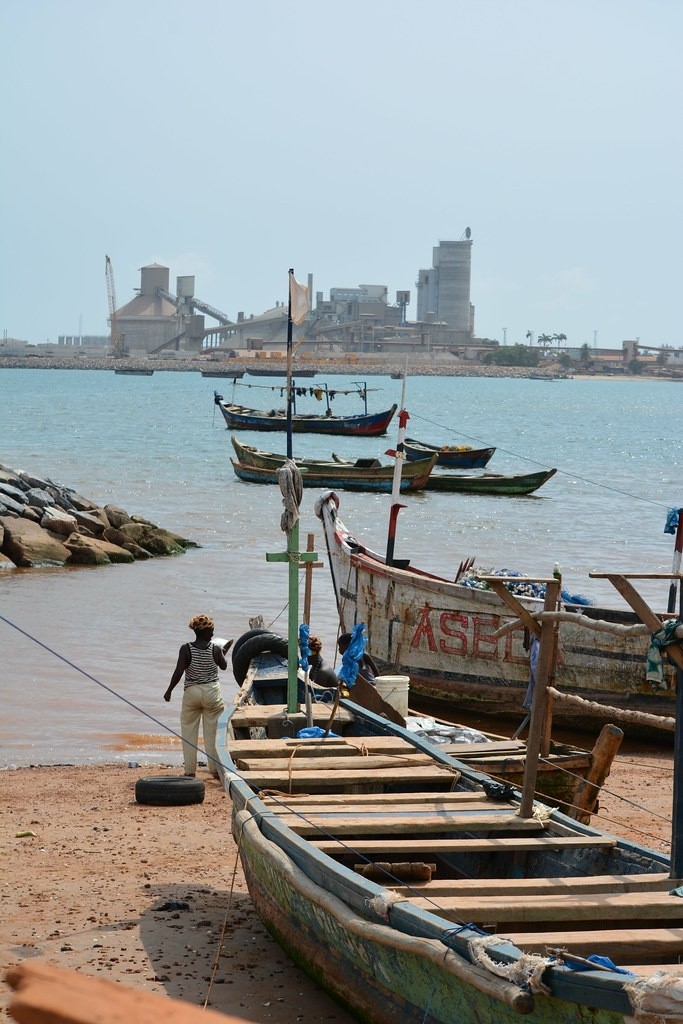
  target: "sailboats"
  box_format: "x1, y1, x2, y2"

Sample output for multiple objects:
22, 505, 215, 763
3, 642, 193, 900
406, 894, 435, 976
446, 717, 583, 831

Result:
230, 268, 440, 491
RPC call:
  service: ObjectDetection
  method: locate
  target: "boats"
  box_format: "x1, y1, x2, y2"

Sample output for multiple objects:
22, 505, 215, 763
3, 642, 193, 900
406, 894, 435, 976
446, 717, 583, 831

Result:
245, 366, 319, 378
330, 451, 556, 497
114, 367, 154, 376
211, 391, 397, 439
229, 457, 420, 493
400, 439, 496, 470
201, 370, 245, 378
529, 375, 553, 380
311, 356, 682, 728
391, 373, 403, 379
228, 269, 682, 1023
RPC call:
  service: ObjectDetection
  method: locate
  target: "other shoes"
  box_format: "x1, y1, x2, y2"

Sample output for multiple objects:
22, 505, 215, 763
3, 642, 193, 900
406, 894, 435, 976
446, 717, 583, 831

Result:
212, 772, 220, 779
180, 772, 195, 778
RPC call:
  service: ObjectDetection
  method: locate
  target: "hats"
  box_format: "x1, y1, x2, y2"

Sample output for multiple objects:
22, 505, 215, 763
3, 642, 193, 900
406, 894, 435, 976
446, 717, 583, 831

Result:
189, 614, 214, 629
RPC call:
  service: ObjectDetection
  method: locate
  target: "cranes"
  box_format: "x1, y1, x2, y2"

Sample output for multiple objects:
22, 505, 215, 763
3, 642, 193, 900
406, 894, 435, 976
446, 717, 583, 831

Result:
291, 319, 320, 364
104, 255, 128, 360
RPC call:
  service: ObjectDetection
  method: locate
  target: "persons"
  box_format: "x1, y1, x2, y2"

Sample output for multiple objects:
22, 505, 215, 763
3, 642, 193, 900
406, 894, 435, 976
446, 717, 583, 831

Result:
298, 635, 338, 687
164, 615, 226, 780
337, 635, 375, 685
